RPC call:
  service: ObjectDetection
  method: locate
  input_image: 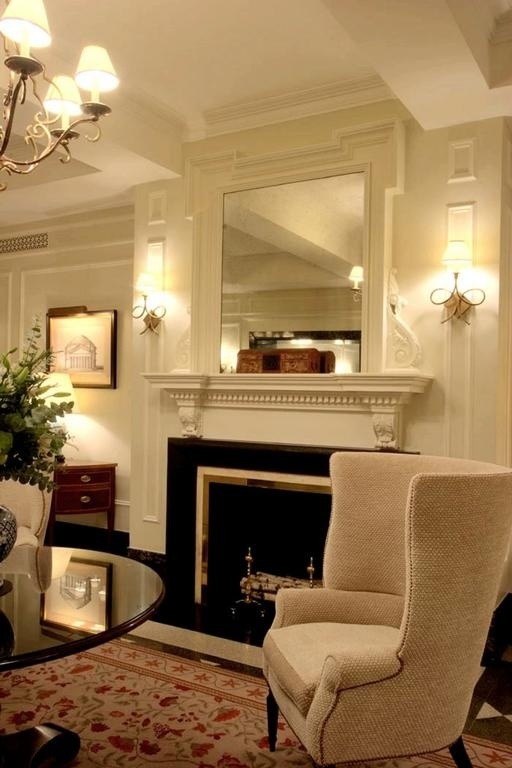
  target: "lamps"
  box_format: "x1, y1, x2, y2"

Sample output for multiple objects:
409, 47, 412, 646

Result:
0, 0, 120, 193
26, 370, 79, 462
51, 547, 73, 578
428, 236, 485, 327
348, 264, 364, 294
130, 268, 168, 334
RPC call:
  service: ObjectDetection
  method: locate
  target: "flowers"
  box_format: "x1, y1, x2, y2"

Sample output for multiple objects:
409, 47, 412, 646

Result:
0, 313, 78, 495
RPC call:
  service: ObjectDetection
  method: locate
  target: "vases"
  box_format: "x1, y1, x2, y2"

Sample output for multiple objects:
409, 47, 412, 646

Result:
0, 501, 17, 565
0, 608, 16, 657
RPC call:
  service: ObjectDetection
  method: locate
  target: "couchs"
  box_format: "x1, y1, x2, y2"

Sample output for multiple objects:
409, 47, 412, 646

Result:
0, 466, 51, 545
0, 545, 52, 593
262, 450, 511, 767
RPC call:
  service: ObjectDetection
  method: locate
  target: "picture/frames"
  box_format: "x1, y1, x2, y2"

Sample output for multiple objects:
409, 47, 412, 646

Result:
38, 556, 114, 637
45, 306, 117, 389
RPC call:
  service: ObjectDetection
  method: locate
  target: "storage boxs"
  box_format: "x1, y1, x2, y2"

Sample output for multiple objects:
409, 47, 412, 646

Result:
236, 349, 278, 373
318, 350, 336, 373
280, 347, 321, 372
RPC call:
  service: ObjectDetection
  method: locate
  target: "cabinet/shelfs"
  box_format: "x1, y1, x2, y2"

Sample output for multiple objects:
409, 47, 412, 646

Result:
51, 458, 119, 543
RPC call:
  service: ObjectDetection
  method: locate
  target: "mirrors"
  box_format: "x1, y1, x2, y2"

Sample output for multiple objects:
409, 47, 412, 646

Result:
220, 171, 366, 374
